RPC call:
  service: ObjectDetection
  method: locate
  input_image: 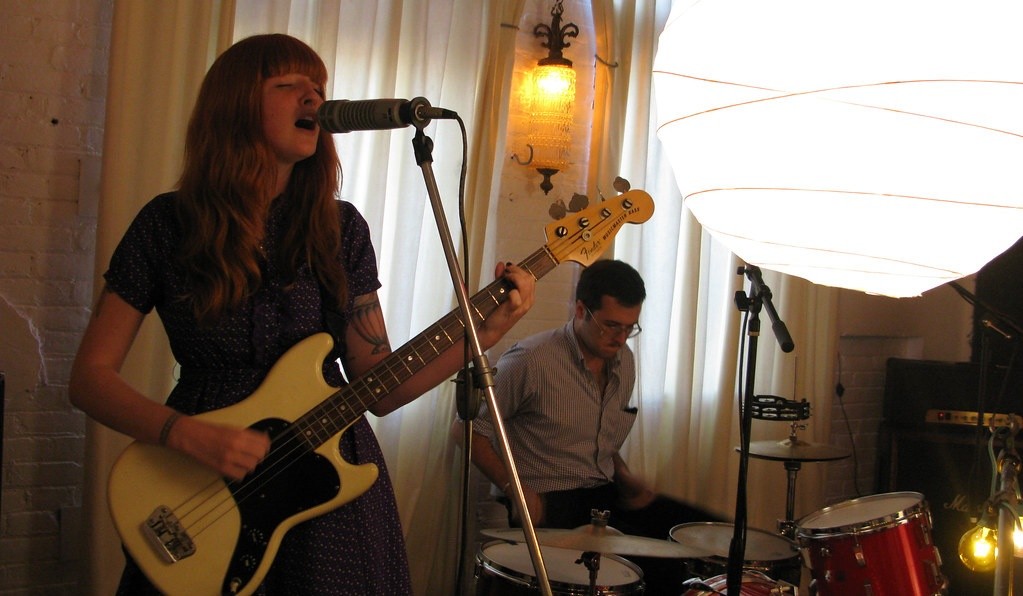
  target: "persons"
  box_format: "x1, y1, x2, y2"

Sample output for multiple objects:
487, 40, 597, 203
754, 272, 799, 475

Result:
68, 34, 537, 595
452, 259, 655, 576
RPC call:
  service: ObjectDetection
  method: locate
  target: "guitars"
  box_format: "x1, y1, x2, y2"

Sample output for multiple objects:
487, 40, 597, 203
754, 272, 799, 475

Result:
103, 173, 656, 596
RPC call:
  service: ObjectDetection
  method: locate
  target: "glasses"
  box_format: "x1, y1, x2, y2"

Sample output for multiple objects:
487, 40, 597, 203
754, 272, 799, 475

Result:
584, 304, 642, 340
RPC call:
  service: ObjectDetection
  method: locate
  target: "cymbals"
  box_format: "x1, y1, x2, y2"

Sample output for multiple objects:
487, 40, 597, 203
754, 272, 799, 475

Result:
479, 522, 715, 559
734, 438, 853, 461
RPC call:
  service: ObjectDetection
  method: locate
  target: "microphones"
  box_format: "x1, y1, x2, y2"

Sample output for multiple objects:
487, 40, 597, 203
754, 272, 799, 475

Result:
316, 97, 457, 133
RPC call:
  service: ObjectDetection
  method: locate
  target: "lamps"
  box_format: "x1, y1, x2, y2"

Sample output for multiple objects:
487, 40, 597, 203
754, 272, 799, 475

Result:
524, 0, 579, 198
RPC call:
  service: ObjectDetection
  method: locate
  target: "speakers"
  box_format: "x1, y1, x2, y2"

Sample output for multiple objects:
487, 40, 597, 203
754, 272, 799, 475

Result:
889, 432, 1023, 596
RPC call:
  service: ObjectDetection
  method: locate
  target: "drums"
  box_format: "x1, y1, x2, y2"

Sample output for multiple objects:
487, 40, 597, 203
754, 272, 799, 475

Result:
471, 490, 953, 596
